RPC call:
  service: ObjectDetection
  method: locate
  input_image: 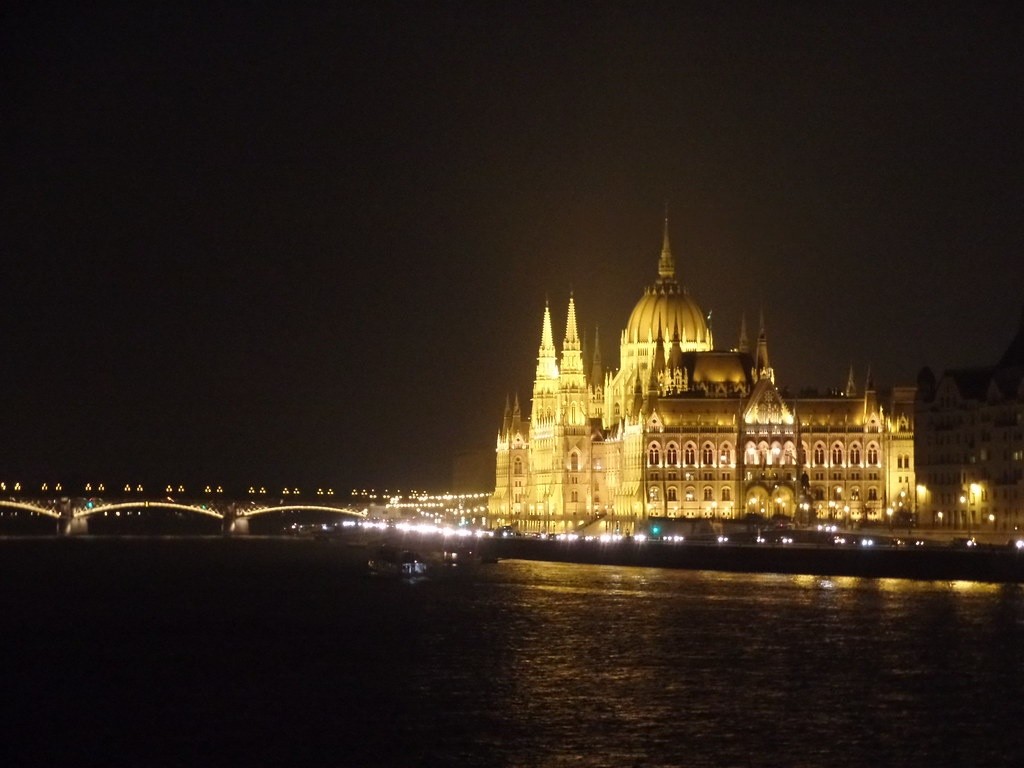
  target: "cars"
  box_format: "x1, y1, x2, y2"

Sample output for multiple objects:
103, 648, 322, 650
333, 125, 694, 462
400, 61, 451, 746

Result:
494, 526, 522, 537
